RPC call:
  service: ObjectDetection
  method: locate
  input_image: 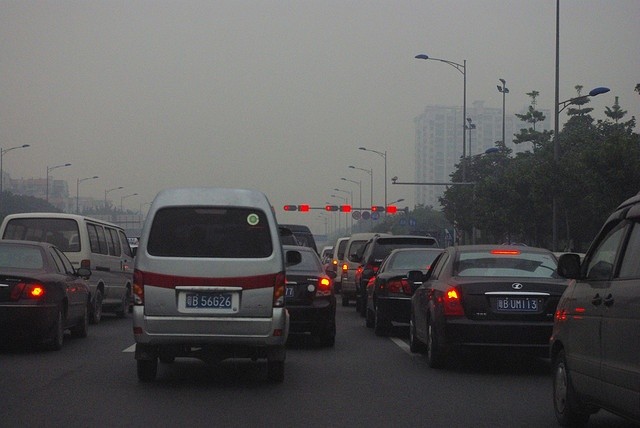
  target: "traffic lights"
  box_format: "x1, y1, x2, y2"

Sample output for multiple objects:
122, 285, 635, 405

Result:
388, 207, 396, 212
341, 206, 351, 212
299, 205, 309, 212
327, 206, 338, 210
372, 207, 384, 211
285, 206, 296, 211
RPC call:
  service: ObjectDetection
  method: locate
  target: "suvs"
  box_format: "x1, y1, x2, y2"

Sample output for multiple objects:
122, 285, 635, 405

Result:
357, 235, 437, 311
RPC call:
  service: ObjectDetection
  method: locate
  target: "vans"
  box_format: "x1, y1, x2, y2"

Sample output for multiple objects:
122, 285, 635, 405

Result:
131, 188, 289, 383
332, 238, 350, 282
321, 247, 332, 260
551, 197, 640, 427
279, 224, 317, 249
1, 213, 134, 322
343, 234, 388, 305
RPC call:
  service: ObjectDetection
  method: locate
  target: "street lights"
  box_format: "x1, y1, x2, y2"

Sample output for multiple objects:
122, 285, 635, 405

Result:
415, 54, 497, 179
334, 189, 354, 211
1, 145, 30, 196
342, 177, 362, 205
77, 176, 98, 212
554, 85, 610, 159
350, 165, 374, 206
359, 147, 405, 206
121, 194, 137, 211
332, 194, 341, 231
46, 163, 71, 201
104, 187, 124, 202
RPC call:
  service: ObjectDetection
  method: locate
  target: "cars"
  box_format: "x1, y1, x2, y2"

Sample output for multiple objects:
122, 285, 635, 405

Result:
365, 248, 442, 337
0, 240, 91, 350
282, 246, 336, 345
408, 244, 567, 369
